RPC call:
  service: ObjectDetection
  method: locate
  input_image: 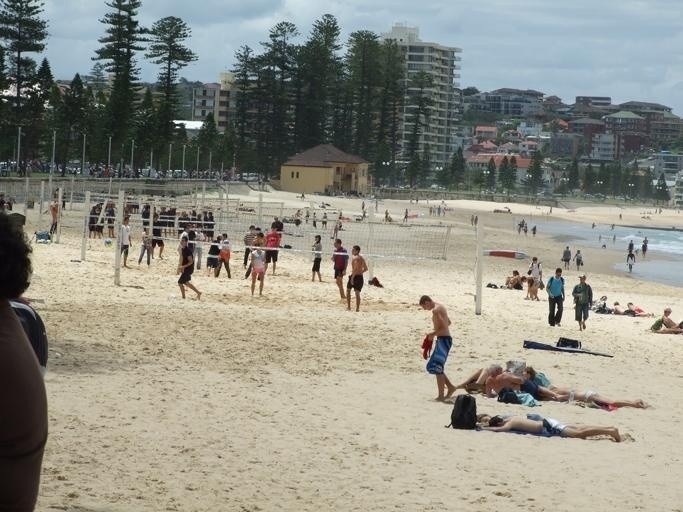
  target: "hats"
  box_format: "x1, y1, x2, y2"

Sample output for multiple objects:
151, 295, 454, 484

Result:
578, 274, 586, 279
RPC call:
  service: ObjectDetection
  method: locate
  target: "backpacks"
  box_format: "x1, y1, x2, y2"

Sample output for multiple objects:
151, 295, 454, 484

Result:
651, 318, 663, 330
445, 393, 478, 430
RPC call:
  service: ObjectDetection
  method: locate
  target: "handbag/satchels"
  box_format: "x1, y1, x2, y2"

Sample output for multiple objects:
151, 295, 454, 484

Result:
557, 336, 581, 350
528, 270, 532, 275
